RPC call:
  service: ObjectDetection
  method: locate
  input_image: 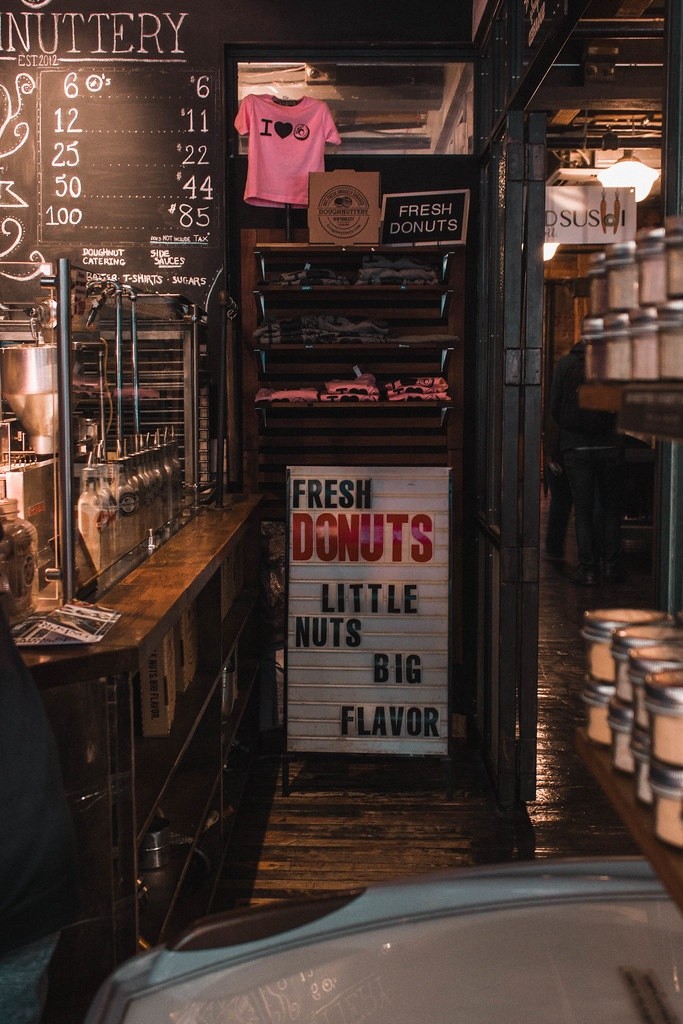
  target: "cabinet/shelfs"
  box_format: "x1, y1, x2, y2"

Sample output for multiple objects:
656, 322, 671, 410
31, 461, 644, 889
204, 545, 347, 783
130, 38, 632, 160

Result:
241, 227, 464, 521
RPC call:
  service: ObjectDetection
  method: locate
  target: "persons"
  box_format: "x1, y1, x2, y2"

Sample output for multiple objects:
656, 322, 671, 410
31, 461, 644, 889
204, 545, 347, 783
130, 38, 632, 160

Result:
545, 315, 627, 587
0, 602, 85, 1024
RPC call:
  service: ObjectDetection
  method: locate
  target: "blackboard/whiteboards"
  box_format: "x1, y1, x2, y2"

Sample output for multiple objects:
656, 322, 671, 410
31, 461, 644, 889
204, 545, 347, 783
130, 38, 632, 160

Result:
0, 0, 224, 304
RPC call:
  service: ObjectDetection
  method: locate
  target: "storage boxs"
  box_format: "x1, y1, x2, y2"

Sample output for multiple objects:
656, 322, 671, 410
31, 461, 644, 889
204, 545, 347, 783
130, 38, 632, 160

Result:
307, 170, 381, 245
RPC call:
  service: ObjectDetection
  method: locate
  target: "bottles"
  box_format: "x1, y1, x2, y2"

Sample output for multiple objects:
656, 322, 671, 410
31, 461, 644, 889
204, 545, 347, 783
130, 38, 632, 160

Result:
0, 497, 42, 626
578, 607, 683, 849
580, 223, 683, 378
76, 426, 184, 592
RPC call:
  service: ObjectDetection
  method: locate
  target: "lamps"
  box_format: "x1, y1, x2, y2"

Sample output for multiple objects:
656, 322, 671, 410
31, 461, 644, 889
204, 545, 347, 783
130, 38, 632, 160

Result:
598, 149, 659, 202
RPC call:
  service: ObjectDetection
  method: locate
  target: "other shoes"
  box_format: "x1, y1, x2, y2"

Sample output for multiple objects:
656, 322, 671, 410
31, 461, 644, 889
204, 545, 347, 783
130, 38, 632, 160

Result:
603, 566, 624, 579
570, 573, 595, 585
